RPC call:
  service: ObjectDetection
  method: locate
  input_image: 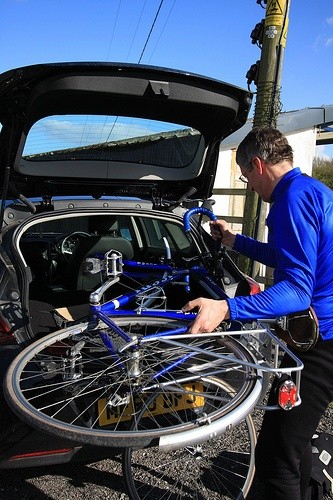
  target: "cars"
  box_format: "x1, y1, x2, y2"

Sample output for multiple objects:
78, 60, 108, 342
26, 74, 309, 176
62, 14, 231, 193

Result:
0, 60, 264, 475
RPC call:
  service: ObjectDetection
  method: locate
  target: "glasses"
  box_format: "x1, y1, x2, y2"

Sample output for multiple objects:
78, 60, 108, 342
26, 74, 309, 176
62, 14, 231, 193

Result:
239, 163, 252, 183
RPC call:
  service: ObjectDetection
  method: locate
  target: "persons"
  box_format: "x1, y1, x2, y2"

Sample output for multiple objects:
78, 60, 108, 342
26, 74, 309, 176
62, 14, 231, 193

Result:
182, 128, 332, 499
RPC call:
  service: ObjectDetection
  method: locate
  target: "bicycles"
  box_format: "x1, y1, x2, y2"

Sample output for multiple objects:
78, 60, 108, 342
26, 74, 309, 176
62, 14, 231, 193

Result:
5, 207, 322, 500
